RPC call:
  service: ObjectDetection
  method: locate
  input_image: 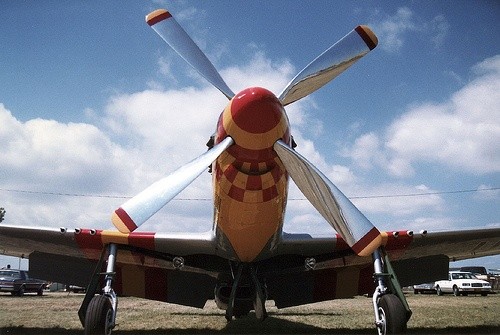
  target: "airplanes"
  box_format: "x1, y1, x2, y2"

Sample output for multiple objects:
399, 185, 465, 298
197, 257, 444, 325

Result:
0, 8, 500, 335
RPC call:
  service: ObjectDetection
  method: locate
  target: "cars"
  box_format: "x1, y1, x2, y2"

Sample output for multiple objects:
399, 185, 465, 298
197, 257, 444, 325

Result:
413, 271, 492, 297
0, 269, 48, 296
